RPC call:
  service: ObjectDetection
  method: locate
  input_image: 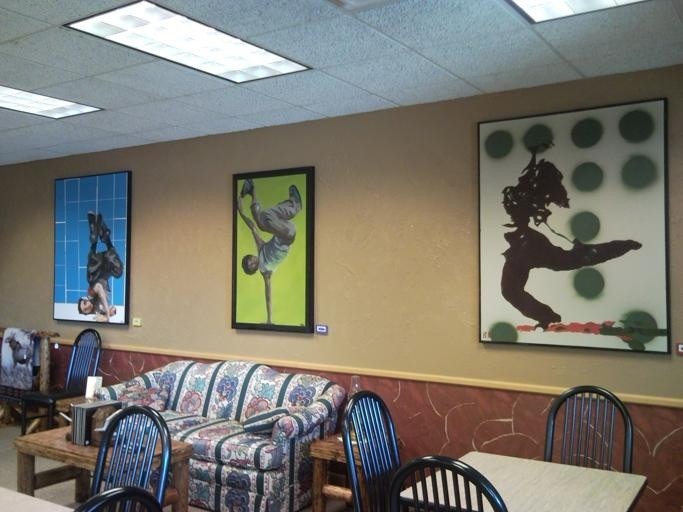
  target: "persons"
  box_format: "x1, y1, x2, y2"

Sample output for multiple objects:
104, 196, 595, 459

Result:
236, 179, 302, 324
77, 210, 122, 322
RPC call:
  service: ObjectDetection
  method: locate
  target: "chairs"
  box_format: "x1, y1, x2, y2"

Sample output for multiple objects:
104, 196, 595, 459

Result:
90, 406, 172, 501
546, 386, 633, 476
341, 389, 404, 512
18, 327, 102, 436
74, 486, 168, 512
387, 456, 508, 512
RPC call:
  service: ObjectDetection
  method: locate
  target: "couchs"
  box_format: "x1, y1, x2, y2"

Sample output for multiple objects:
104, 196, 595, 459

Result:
100, 361, 346, 512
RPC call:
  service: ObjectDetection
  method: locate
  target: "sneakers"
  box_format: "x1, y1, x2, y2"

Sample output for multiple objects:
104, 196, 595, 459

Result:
97, 213, 106, 235
87, 211, 96, 229
240, 178, 252, 200
288, 185, 302, 210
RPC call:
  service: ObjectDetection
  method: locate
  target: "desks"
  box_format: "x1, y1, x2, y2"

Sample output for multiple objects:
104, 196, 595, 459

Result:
15, 425, 193, 512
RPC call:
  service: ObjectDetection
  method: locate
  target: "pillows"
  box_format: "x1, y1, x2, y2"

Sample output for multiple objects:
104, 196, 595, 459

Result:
120, 386, 169, 415
245, 405, 305, 432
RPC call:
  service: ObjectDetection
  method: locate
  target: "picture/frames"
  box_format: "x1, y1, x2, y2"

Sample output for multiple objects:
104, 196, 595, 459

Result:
474, 97, 672, 354
228, 166, 315, 334
52, 171, 133, 326
